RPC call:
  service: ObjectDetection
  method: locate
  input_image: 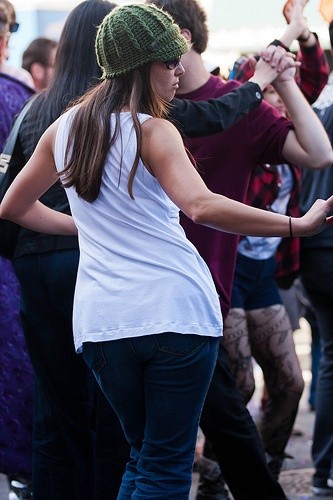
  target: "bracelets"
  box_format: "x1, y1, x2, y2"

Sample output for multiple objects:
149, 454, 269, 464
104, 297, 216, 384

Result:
298, 33, 310, 42
289, 216, 294, 238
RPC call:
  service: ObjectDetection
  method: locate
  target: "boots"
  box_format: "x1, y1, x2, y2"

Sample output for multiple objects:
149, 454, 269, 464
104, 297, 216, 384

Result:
193, 457, 230, 500
264, 451, 294, 482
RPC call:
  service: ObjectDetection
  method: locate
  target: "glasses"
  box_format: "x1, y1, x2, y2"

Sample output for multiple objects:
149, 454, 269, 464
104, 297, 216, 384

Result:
4, 21, 19, 33
163, 55, 182, 70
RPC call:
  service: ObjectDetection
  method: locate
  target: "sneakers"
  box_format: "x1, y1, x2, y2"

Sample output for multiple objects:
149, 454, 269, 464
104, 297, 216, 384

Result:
312, 483, 333, 494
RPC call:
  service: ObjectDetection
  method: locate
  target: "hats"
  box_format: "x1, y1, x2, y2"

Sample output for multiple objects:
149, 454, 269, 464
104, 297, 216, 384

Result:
96, 5, 188, 79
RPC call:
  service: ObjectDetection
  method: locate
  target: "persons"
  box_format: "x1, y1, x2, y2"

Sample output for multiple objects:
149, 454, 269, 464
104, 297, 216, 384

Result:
0, 3, 333, 500
0, 0, 333, 500
